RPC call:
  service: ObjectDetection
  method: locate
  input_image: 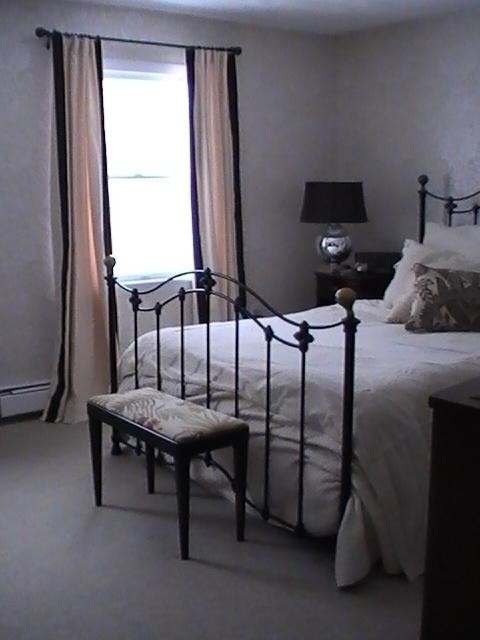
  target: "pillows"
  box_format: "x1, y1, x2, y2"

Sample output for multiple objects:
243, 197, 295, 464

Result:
404, 263, 480, 332
422, 222, 480, 261
384, 239, 444, 311
385, 251, 480, 323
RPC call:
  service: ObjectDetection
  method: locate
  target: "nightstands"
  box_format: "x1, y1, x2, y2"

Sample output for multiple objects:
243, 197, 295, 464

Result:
316, 271, 395, 306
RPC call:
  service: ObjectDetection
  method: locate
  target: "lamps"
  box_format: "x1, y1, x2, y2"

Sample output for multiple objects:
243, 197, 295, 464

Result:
298, 182, 368, 273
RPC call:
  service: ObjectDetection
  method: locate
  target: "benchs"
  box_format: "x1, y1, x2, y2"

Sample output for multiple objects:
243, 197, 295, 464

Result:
87, 387, 249, 559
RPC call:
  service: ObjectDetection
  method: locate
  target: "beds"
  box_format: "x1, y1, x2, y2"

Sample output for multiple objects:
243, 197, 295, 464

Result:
101, 173, 480, 591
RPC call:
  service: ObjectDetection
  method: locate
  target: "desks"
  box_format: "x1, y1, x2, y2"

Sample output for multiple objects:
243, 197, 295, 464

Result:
423, 375, 479, 638
